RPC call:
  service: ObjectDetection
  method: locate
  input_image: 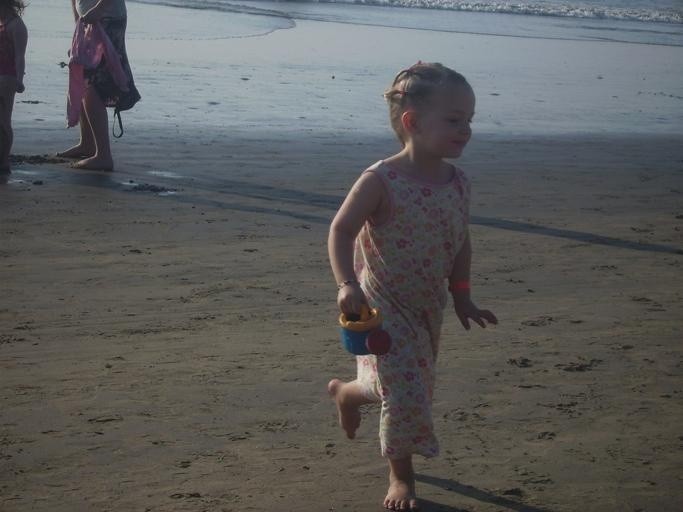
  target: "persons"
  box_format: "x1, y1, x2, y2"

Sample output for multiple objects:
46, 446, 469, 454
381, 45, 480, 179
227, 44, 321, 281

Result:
54, 0, 141, 171
321, 55, 498, 512
0, 0, 28, 174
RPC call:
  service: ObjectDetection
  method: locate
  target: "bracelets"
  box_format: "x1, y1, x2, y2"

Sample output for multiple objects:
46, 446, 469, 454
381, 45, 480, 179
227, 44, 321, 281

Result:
446, 281, 471, 293
334, 278, 363, 293
